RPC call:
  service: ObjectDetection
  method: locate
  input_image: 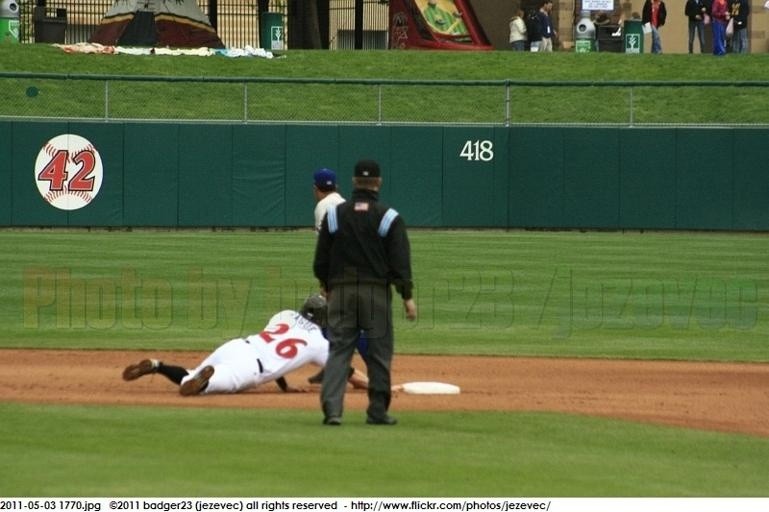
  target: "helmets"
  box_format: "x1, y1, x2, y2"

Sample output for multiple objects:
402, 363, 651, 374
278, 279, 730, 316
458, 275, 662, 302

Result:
301, 295, 327, 329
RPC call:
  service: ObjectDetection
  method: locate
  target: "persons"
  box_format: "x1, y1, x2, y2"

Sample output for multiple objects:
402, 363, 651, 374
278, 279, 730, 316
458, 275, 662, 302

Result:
310, 160, 417, 428
505, 0, 756, 57
121, 291, 406, 398
309, 167, 373, 389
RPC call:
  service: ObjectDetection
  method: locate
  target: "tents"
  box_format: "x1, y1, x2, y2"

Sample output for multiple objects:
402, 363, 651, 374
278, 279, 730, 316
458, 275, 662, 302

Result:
88, 0, 228, 52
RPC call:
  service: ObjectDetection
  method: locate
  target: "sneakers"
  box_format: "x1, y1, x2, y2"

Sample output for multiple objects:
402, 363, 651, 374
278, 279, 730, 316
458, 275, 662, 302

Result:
123, 359, 159, 381
180, 366, 214, 397
324, 416, 341, 425
366, 416, 396, 425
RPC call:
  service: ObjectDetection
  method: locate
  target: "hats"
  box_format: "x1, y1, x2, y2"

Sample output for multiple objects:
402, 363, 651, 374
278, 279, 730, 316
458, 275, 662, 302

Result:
314, 168, 336, 186
354, 160, 380, 177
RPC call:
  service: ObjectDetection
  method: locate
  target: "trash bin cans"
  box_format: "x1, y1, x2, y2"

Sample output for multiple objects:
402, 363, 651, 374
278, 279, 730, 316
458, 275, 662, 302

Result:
623, 19, 643, 54
260, 11, 285, 51
32, 6, 68, 44
